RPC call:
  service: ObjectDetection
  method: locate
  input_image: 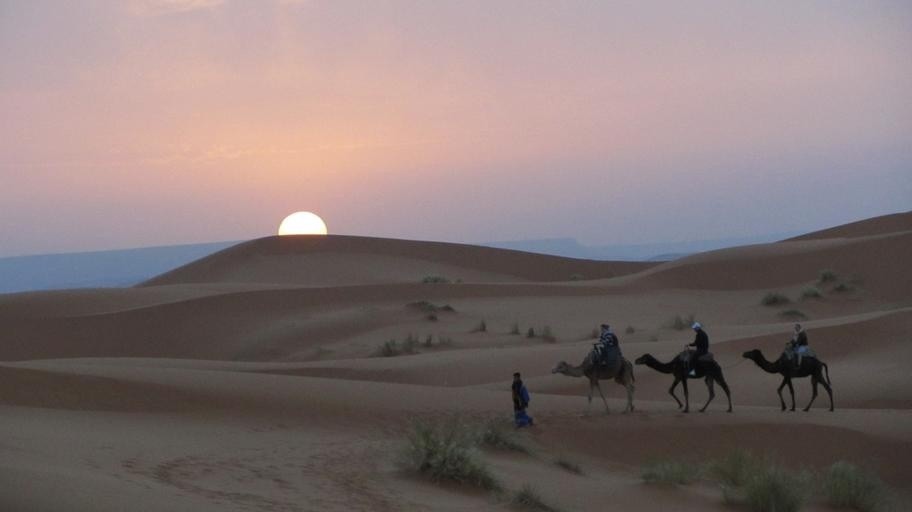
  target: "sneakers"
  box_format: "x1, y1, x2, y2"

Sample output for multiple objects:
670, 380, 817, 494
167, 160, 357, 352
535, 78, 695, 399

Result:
689, 369, 695, 375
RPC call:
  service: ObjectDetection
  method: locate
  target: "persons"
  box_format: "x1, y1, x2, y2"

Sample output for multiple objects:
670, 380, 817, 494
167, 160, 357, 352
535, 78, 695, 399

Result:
688, 322, 709, 376
512, 372, 533, 430
787, 323, 809, 370
594, 324, 618, 367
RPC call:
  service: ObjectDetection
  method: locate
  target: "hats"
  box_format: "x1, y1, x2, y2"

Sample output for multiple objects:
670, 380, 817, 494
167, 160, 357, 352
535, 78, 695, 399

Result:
692, 323, 701, 329
